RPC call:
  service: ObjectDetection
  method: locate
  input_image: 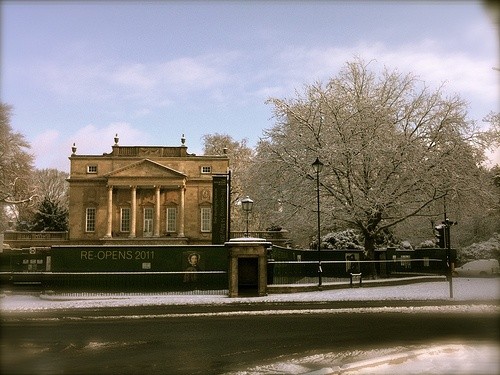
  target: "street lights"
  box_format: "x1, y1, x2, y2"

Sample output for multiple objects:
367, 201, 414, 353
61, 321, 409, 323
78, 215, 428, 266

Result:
241, 195, 254, 238
312, 155, 327, 291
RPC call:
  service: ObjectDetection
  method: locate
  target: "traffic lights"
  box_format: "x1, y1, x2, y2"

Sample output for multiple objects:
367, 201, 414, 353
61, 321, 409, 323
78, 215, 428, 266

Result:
434, 224, 444, 248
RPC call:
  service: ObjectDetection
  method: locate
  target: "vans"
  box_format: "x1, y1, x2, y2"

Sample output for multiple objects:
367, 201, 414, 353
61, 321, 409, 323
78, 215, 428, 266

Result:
453, 259, 499, 277
